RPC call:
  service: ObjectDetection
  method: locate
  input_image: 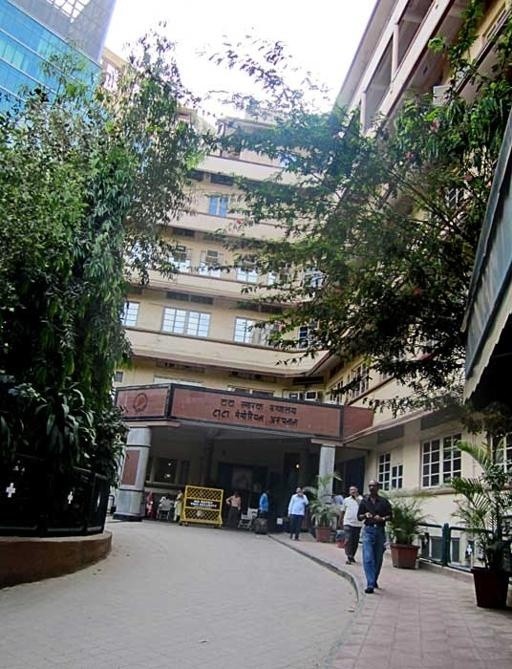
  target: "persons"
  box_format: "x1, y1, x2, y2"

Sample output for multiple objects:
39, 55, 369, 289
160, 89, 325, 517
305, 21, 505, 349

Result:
172, 488, 184, 522
259, 487, 269, 518
357, 480, 393, 593
338, 485, 363, 564
146, 492, 154, 519
225, 491, 243, 527
287, 486, 310, 540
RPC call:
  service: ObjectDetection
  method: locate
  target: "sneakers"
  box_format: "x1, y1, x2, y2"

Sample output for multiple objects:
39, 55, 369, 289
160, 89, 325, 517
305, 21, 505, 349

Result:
363, 582, 379, 594
346, 556, 356, 565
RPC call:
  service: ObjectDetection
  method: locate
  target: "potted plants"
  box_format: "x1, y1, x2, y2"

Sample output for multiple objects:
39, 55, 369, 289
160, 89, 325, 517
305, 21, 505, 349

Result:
451, 434, 512, 611
299, 467, 347, 544
377, 482, 435, 571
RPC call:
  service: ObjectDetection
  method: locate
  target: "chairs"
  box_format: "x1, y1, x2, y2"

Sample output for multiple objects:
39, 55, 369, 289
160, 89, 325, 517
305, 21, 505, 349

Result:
237, 505, 259, 531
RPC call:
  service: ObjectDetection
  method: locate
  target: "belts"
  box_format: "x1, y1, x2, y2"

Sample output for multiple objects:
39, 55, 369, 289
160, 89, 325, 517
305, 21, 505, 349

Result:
365, 523, 384, 527
